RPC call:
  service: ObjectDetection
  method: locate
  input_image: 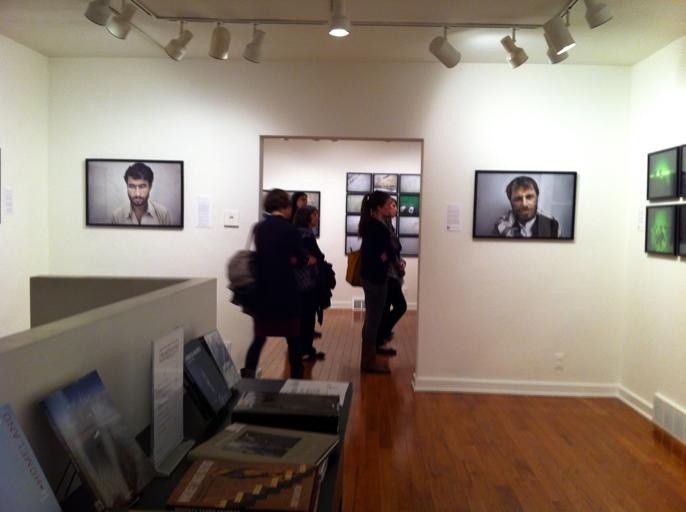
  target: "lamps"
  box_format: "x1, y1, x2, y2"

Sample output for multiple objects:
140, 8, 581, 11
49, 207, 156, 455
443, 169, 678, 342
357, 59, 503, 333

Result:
326, 0, 353, 39
82, 0, 140, 42
542, 0, 615, 56
426, 26, 571, 70
162, 18, 269, 66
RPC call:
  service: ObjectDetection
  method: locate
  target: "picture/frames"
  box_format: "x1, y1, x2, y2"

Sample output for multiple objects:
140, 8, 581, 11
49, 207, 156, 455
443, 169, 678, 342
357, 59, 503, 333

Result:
263, 188, 322, 239
83, 157, 186, 230
471, 169, 578, 241
344, 171, 421, 258
646, 144, 684, 202
644, 200, 681, 260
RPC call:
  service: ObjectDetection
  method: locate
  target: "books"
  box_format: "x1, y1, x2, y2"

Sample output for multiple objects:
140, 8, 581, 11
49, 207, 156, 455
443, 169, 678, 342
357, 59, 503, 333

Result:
1, 403, 63, 512
180, 330, 240, 421
186, 422, 340, 467
166, 456, 322, 512
38, 369, 155, 512
227, 388, 340, 433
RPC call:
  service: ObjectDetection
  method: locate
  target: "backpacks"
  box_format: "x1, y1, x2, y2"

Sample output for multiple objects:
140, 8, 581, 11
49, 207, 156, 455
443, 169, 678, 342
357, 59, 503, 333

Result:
228, 250, 258, 290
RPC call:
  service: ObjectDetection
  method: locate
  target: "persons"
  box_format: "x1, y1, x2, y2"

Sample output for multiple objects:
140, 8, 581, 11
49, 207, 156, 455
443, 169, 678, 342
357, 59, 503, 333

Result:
491, 176, 558, 237
377, 196, 407, 357
296, 205, 327, 361
111, 162, 171, 226
358, 190, 392, 373
291, 191, 307, 226
241, 187, 316, 379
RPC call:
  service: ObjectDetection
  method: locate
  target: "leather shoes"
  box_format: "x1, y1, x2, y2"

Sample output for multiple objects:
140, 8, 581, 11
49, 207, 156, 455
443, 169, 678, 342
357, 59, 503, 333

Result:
314, 332, 322, 338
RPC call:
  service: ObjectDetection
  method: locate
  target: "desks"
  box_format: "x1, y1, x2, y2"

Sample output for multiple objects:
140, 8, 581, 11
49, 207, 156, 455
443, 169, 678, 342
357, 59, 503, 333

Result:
57, 377, 357, 511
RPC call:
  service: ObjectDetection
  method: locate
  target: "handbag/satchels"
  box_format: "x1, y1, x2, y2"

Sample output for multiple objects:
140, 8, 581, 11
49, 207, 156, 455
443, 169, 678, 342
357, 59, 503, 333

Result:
345, 248, 364, 287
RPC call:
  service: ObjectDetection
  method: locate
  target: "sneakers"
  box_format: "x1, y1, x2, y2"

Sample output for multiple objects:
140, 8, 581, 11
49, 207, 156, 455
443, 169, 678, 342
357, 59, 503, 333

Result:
241, 368, 254, 379
300, 346, 325, 364
361, 349, 397, 374
